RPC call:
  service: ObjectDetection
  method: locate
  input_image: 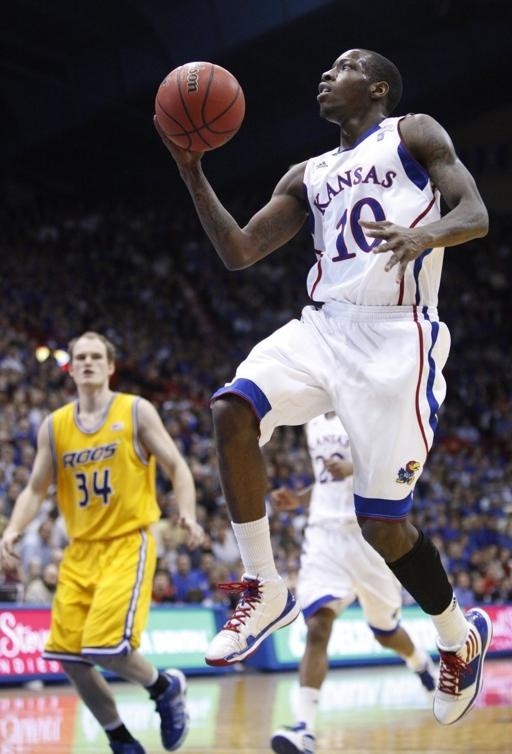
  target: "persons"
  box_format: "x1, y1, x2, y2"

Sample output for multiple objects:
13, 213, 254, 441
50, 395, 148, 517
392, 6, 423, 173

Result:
153, 47, 493, 724
1, 331, 198, 754
1, 180, 512, 602
269, 413, 440, 754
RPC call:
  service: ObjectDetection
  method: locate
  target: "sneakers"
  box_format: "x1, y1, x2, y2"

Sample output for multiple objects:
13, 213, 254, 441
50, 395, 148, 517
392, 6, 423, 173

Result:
271, 721, 315, 754
205, 572, 301, 667
413, 607, 493, 728
150, 668, 191, 752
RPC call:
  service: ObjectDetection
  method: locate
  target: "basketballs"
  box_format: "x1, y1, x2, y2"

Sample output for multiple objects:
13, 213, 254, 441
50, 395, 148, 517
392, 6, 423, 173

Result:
156, 61, 245, 148
